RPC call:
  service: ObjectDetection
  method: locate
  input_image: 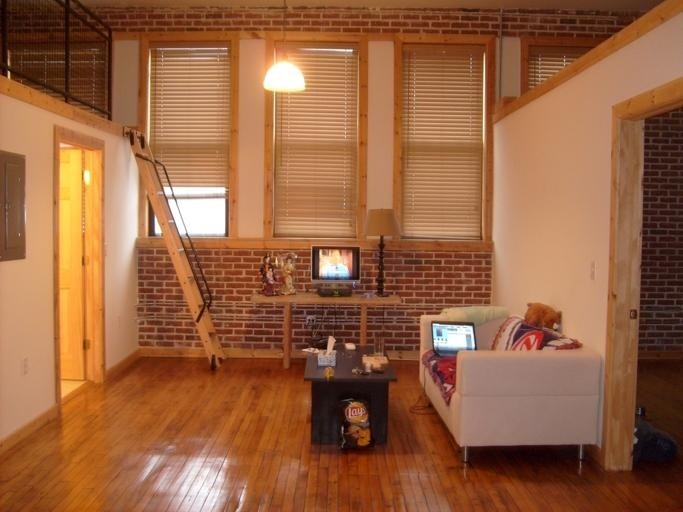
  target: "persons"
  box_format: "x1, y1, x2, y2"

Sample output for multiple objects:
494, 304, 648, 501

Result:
279, 254, 295, 295
258, 253, 278, 296
320, 250, 349, 278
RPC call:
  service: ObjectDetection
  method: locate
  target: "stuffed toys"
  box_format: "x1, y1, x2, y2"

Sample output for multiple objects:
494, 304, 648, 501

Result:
524, 303, 561, 331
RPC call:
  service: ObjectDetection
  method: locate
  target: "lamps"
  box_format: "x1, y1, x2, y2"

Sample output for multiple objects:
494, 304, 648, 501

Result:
363, 209, 404, 297
262, 0, 305, 93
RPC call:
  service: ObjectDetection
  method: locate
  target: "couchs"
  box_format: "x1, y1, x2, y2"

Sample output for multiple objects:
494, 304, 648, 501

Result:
418, 306, 602, 469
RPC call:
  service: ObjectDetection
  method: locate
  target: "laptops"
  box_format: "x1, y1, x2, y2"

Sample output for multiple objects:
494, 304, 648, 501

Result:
430, 321, 479, 358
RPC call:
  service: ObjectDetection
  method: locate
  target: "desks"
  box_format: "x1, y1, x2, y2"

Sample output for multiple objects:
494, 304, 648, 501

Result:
250, 292, 402, 369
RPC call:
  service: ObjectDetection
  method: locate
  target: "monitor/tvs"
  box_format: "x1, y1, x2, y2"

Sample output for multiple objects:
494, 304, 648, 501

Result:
309, 245, 361, 290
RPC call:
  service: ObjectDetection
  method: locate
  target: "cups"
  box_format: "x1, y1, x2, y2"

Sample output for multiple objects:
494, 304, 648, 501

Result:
364, 292, 378, 300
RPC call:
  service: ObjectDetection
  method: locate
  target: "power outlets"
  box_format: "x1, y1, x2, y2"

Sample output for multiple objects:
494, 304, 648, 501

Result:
305, 315, 317, 326
